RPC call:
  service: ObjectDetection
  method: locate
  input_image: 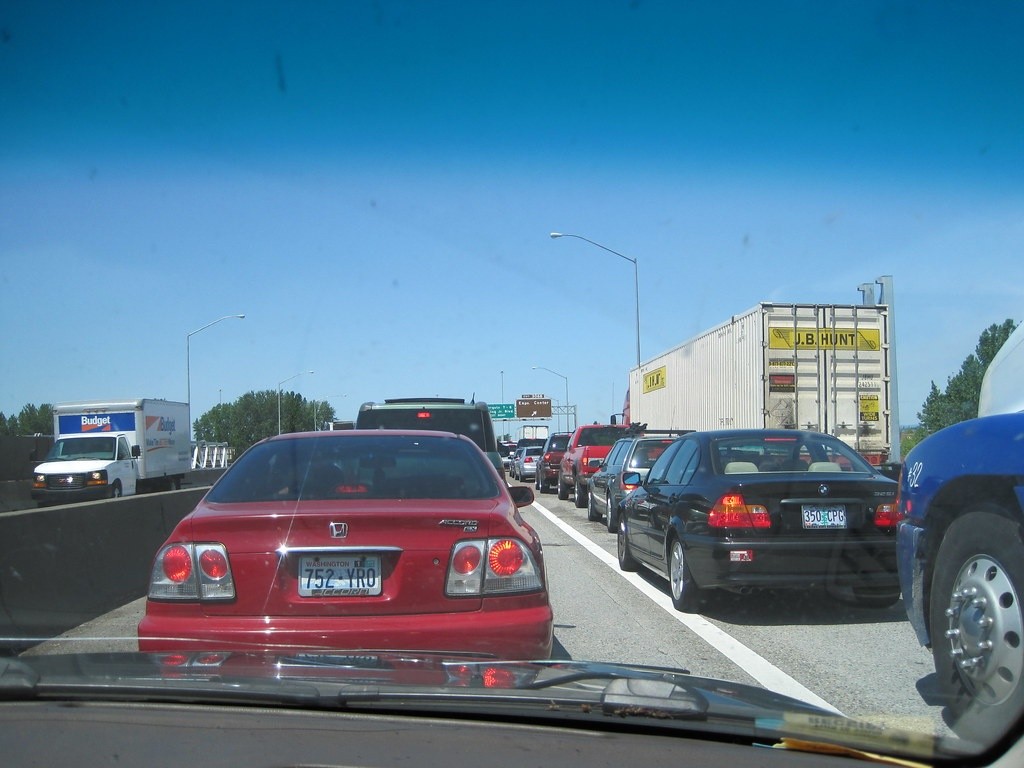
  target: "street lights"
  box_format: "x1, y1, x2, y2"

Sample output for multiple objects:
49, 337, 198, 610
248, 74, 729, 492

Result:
314, 393, 348, 431
278, 370, 314, 434
500, 370, 504, 403
551, 232, 641, 367
184, 313, 246, 433
532, 367, 570, 432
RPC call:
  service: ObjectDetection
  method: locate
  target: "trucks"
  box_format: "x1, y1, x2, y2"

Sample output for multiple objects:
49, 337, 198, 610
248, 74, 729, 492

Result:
898, 319, 1024, 750
31, 397, 192, 507
610, 299, 891, 476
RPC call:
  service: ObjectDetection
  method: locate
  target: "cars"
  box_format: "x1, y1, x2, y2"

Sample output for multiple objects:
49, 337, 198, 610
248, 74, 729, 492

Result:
497, 423, 697, 535
137, 429, 554, 693
616, 428, 912, 615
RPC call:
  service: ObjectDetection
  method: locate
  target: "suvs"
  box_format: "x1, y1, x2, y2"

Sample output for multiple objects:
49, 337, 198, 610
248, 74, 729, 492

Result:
353, 395, 509, 500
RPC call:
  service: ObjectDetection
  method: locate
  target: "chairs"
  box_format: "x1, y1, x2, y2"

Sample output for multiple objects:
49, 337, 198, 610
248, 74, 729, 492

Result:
725, 459, 842, 473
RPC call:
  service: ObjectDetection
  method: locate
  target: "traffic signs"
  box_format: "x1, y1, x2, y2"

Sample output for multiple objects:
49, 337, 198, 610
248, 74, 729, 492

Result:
517, 399, 551, 418
